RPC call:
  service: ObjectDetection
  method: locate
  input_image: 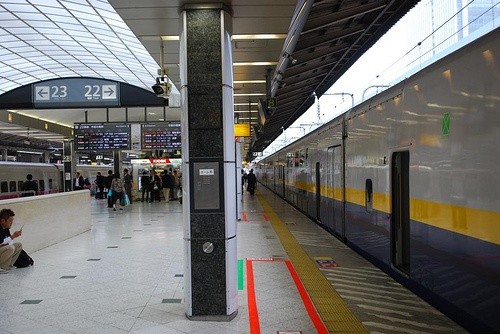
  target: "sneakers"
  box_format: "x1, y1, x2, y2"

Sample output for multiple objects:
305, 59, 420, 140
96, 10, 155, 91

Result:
0, 266, 16, 274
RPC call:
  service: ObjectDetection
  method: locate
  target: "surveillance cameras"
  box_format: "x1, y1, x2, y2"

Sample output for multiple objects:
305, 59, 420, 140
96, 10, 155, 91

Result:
152, 82, 171, 95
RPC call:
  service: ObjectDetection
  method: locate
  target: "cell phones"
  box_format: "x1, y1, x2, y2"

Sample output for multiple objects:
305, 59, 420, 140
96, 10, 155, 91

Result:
20, 225, 24, 232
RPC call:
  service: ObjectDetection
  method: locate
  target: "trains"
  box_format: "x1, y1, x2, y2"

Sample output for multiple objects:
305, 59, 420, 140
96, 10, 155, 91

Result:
1, 156, 182, 196
248, 24, 500, 333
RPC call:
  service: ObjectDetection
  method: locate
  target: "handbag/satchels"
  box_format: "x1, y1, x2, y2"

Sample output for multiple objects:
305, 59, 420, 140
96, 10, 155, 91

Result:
124, 194, 130, 206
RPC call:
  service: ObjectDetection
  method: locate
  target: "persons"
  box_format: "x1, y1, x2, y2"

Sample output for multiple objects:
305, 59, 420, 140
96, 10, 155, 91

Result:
241, 169, 258, 199
20, 175, 38, 197
0, 208, 23, 274
73, 172, 85, 191
95, 167, 182, 211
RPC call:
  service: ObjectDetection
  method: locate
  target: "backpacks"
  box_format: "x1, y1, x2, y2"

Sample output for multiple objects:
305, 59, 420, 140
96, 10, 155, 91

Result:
14, 249, 34, 268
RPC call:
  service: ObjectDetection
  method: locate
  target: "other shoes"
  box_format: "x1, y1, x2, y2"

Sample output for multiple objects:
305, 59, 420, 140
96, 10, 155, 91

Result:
141, 200, 148, 203
113, 208, 117, 212
149, 200, 154, 203
158, 200, 161, 202
165, 201, 169, 203
119, 208, 123, 210
